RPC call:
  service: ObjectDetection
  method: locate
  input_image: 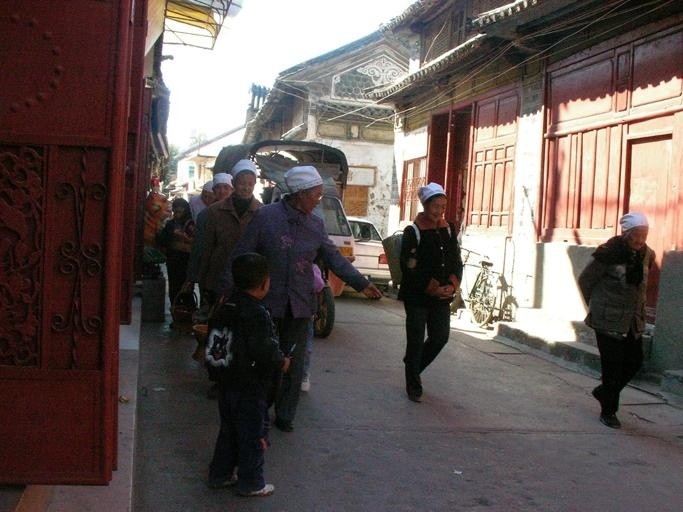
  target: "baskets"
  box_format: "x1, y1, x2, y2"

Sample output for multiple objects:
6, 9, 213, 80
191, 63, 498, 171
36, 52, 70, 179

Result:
171, 219, 194, 253
170, 289, 200, 327
382, 234, 403, 285
193, 323, 210, 346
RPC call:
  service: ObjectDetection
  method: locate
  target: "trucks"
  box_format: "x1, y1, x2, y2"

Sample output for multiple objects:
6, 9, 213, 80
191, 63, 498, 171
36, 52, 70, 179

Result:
211, 139, 355, 338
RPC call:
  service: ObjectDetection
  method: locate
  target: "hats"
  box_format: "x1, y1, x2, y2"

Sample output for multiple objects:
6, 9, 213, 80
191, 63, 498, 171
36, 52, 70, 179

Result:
203, 173, 233, 193
231, 159, 258, 177
619, 212, 648, 232
285, 165, 324, 194
418, 182, 446, 202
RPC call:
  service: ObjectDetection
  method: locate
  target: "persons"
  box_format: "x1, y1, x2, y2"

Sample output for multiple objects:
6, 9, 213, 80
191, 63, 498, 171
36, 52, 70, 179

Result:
578, 212, 656, 429
160, 159, 383, 497
397, 181, 463, 401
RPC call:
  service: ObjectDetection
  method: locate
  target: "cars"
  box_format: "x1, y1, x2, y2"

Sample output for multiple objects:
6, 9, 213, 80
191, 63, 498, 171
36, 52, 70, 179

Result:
344, 217, 391, 301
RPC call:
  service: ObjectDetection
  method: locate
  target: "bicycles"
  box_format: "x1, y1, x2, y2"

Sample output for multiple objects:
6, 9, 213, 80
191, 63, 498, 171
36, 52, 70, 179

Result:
450, 245, 505, 329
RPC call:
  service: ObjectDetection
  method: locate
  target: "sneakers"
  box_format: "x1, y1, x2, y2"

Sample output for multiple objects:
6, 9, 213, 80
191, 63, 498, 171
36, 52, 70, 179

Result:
599, 412, 621, 428
274, 417, 295, 432
408, 389, 424, 401
300, 375, 310, 392
242, 484, 275, 497
